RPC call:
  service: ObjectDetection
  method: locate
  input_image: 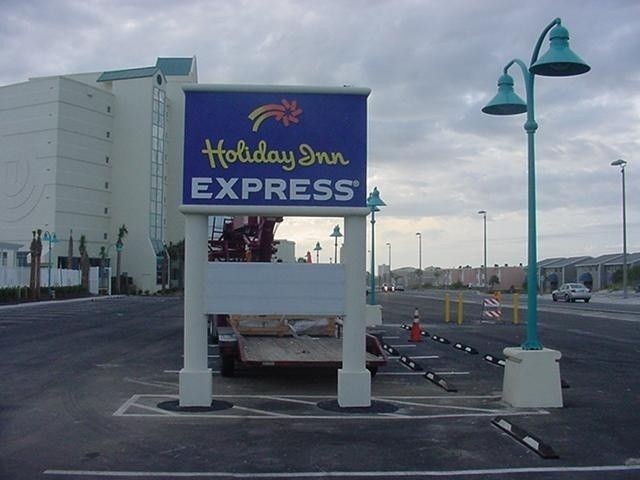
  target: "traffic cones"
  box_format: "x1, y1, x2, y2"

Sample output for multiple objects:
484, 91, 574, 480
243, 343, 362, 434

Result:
408, 307, 423, 343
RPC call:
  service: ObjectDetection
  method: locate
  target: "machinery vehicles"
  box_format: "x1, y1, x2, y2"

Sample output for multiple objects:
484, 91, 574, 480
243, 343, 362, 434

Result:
208, 214, 387, 387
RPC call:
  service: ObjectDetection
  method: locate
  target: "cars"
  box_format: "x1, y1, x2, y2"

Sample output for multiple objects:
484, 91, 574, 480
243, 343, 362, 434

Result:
551, 283, 591, 303
365, 282, 404, 294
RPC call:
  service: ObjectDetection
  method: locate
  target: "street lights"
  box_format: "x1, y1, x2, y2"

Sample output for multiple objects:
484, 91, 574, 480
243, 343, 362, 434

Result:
314, 241, 322, 264
478, 210, 487, 294
415, 232, 422, 292
366, 186, 387, 327
329, 223, 343, 264
481, 17, 591, 409
102, 242, 125, 294
386, 242, 391, 282
42, 231, 60, 300
610, 158, 628, 299
